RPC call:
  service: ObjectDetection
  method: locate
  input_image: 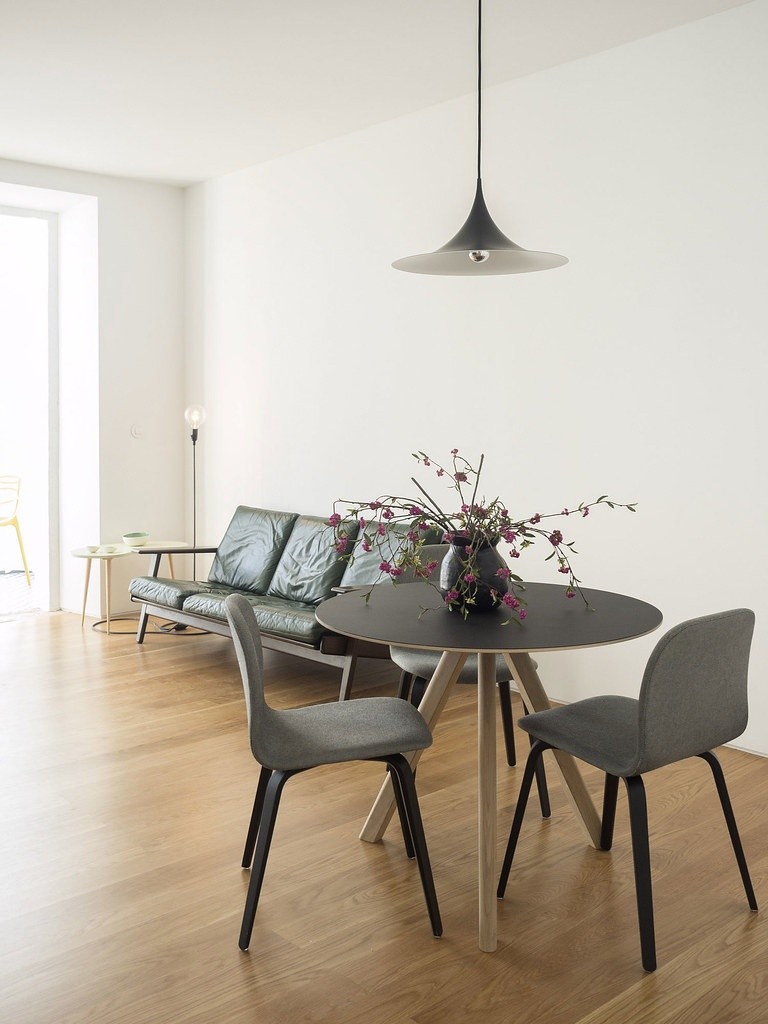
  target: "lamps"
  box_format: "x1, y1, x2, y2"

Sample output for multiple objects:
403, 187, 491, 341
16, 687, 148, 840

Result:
390, 0, 569, 276
185, 404, 206, 580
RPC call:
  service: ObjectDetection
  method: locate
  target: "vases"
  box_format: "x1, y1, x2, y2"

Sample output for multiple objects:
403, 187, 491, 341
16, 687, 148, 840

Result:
439, 528, 514, 617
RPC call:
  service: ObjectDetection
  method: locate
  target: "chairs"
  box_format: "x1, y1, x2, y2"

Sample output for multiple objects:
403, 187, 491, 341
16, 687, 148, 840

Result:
0, 475, 31, 586
224, 594, 443, 952
497, 609, 758, 972
387, 543, 538, 774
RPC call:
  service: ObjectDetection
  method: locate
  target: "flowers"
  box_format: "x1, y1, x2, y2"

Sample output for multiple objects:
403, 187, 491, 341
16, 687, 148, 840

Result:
320, 448, 639, 620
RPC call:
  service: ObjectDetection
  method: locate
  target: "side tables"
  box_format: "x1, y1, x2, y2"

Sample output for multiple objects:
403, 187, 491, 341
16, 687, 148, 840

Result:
73, 541, 188, 636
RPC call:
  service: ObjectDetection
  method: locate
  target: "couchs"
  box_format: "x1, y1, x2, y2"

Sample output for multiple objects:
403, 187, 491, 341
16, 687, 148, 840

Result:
136, 506, 470, 700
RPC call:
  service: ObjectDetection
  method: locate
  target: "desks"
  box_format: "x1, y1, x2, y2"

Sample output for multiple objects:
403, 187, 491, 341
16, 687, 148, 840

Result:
314, 580, 663, 952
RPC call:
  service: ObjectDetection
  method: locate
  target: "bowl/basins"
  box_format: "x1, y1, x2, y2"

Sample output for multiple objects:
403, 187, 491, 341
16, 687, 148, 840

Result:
87, 546, 100, 553
104, 547, 117, 553
122, 531, 150, 545
